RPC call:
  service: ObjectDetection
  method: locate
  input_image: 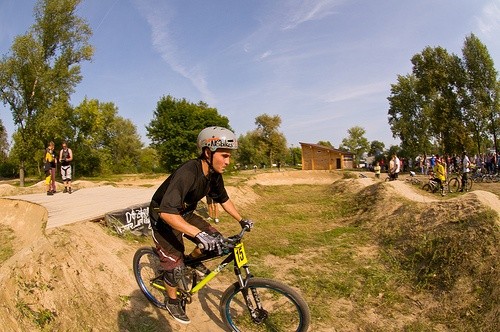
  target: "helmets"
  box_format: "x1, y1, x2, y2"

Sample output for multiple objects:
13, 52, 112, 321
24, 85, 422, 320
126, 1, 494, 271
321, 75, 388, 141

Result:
197, 126, 238, 154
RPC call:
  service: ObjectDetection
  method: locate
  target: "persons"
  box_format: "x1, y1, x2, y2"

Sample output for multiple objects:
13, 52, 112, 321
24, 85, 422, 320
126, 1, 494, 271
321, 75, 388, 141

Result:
206, 195, 219, 223
149, 126, 253, 324
368, 150, 500, 196
43, 141, 73, 195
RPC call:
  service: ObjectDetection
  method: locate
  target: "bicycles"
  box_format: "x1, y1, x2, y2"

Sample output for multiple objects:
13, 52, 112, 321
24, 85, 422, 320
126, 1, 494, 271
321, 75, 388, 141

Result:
132, 224, 309, 332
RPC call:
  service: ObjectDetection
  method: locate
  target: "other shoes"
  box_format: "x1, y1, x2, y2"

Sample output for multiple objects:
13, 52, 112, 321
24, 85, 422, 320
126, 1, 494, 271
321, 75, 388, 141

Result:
63, 188, 67, 193
69, 190, 71, 194
206, 216, 213, 222
442, 191, 446, 196
47, 190, 54, 195
214, 218, 219, 225
52, 191, 56, 193
459, 190, 465, 192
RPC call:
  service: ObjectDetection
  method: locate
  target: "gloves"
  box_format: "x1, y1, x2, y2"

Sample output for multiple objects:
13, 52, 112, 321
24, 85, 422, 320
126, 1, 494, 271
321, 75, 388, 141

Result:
194, 232, 224, 257
239, 218, 254, 232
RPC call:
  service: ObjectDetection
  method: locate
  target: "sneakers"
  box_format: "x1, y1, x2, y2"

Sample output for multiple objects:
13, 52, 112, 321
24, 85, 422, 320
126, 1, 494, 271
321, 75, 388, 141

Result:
185, 255, 211, 279
166, 299, 191, 323
